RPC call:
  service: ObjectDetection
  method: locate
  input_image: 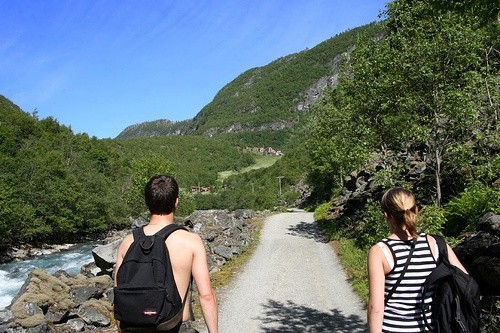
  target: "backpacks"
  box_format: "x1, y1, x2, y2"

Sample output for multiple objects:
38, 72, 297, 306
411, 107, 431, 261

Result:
422, 235, 480, 333
114, 225, 189, 330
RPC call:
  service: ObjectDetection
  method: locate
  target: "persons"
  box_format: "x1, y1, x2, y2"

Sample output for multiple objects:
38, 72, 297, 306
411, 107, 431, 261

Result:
368, 187, 481, 333
113, 175, 220, 333
